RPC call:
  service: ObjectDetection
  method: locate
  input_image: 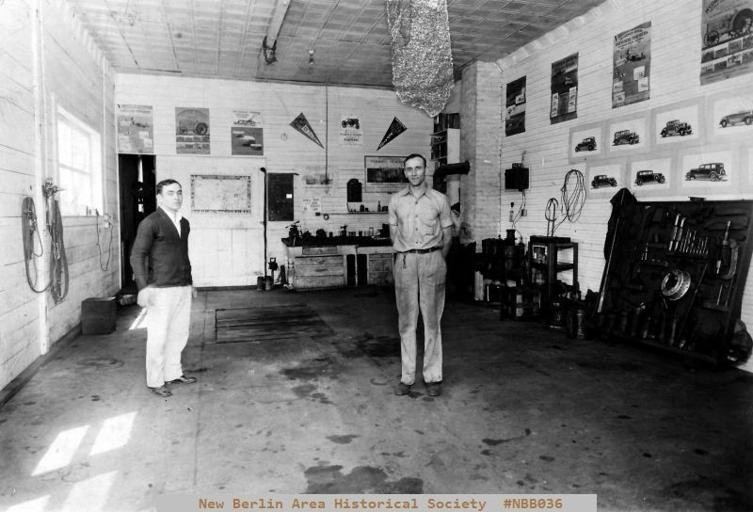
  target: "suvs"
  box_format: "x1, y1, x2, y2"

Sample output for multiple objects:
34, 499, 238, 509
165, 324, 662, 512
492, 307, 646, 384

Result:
685, 162, 726, 181
591, 175, 618, 189
612, 130, 639, 145
634, 170, 665, 185
659, 120, 692, 137
574, 136, 596, 152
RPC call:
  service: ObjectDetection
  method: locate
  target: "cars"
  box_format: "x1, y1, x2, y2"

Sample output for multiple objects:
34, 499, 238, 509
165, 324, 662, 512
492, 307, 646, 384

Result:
719, 110, 752, 127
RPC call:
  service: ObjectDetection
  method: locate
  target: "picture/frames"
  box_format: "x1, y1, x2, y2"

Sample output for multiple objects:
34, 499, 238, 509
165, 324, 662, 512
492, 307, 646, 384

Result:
190, 174, 252, 214
626, 153, 678, 196
705, 85, 752, 143
605, 110, 650, 159
568, 121, 606, 163
584, 157, 627, 198
677, 145, 741, 196
651, 96, 705, 152
363, 154, 407, 194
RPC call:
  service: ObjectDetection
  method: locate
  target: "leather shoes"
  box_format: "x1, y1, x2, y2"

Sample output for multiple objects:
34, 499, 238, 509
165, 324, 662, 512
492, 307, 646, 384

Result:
147, 385, 172, 398
395, 383, 411, 395
426, 381, 441, 396
166, 375, 197, 384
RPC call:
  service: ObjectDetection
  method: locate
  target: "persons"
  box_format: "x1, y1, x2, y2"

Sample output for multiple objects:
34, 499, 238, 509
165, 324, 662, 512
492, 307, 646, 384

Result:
129, 178, 197, 397
387, 153, 452, 396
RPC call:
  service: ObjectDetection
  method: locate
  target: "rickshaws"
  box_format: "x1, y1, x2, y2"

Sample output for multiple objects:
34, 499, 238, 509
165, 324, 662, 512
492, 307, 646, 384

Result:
702, 8, 753, 48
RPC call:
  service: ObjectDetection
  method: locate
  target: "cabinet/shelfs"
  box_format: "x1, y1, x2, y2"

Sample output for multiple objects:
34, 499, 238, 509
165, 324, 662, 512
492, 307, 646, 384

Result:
281, 237, 394, 290
525, 242, 579, 326
430, 128, 461, 205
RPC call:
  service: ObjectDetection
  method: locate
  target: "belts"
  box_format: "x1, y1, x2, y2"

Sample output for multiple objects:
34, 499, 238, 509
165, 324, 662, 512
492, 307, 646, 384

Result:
402, 247, 442, 254
